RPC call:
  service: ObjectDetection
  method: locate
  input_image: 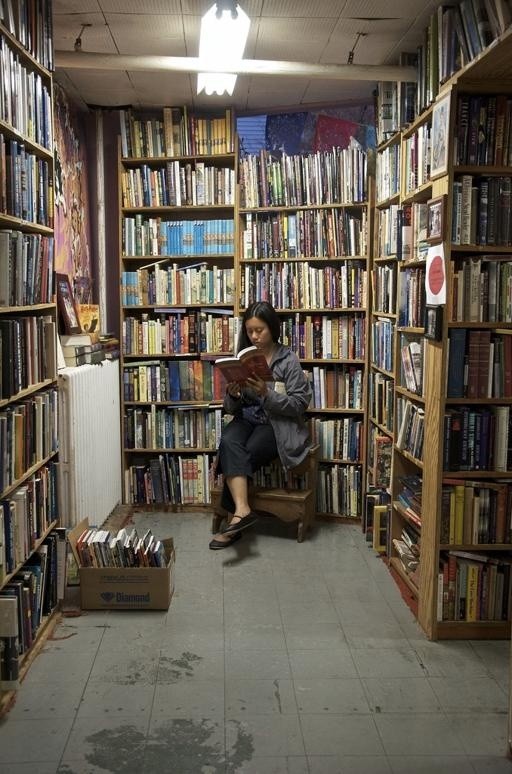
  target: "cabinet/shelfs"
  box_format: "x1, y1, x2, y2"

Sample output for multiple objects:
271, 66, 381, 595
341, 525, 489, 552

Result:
0, 0, 59, 714
364, 86, 510, 641
117, 133, 372, 526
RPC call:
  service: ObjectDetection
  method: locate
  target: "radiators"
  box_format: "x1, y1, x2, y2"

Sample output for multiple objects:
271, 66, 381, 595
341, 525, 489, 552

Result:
61, 352, 124, 565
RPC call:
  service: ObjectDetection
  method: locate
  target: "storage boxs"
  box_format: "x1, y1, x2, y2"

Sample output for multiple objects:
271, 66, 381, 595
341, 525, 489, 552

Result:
62, 516, 177, 611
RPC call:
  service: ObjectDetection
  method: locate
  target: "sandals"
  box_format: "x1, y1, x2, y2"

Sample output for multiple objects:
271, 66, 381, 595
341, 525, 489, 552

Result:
209, 508, 259, 549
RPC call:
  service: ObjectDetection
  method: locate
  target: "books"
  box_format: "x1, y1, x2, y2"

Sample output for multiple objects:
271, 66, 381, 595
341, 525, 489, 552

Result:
117, 0, 512, 623
68, 517, 166, 567
0, 0, 66, 684
60, 304, 120, 367
